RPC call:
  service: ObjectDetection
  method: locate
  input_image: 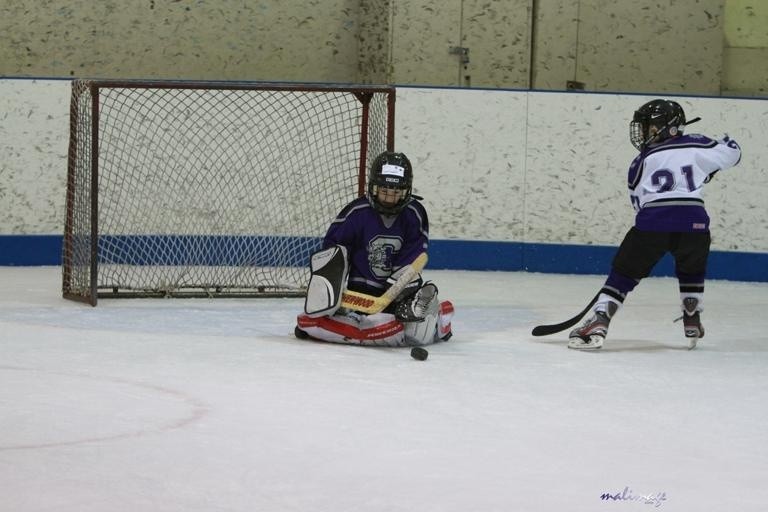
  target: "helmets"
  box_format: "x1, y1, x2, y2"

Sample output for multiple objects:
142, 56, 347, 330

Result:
629, 99, 686, 151
364, 151, 413, 215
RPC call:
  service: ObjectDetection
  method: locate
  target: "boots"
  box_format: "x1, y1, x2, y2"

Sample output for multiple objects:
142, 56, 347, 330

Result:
683, 297, 705, 338
570, 301, 618, 341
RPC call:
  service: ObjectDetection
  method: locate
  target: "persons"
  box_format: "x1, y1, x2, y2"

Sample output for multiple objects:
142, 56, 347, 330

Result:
324, 151, 440, 321
557, 100, 743, 354
